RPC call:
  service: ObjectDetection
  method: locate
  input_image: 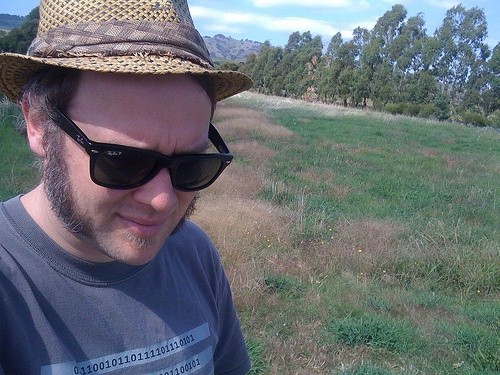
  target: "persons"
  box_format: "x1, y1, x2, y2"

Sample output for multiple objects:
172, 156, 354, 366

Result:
0, 1, 254, 375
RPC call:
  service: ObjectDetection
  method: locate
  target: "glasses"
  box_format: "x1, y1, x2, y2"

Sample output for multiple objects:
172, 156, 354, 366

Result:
42, 98, 234, 192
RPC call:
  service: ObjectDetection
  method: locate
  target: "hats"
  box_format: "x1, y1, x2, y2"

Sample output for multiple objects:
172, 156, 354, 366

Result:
0, 0, 253, 103
